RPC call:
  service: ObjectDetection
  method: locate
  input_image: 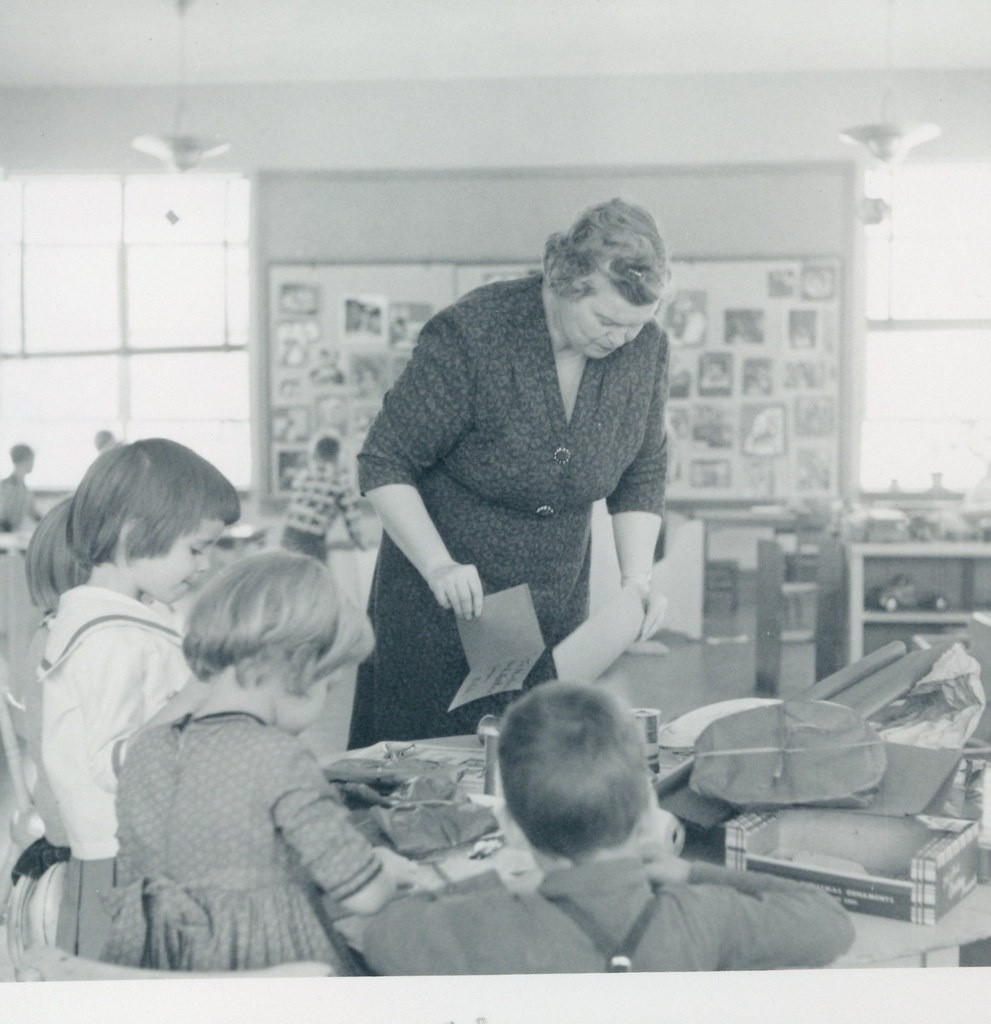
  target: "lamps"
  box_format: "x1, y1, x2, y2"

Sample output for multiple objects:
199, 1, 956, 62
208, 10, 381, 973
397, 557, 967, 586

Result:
838, 42, 940, 160
128, 3, 226, 172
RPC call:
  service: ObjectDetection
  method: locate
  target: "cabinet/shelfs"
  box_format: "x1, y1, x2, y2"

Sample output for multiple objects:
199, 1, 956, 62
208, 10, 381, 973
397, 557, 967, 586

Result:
658, 493, 991, 724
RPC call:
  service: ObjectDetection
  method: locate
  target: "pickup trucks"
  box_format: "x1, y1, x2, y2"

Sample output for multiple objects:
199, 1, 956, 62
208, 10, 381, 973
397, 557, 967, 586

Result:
874, 574, 952, 612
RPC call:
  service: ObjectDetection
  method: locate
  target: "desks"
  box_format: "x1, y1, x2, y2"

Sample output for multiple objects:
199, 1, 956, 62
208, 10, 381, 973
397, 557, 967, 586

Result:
321, 726, 991, 968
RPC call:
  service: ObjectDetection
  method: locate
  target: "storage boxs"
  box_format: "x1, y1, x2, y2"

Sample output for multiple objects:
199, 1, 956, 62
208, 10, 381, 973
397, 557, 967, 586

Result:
724, 805, 980, 927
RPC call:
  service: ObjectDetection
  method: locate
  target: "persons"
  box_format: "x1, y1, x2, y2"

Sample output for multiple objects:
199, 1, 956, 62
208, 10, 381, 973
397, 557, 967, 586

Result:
38, 436, 241, 962
0, 445, 42, 553
98, 551, 419, 976
344, 196, 668, 750
281, 438, 366, 564
364, 680, 857, 977
11, 496, 91, 884
